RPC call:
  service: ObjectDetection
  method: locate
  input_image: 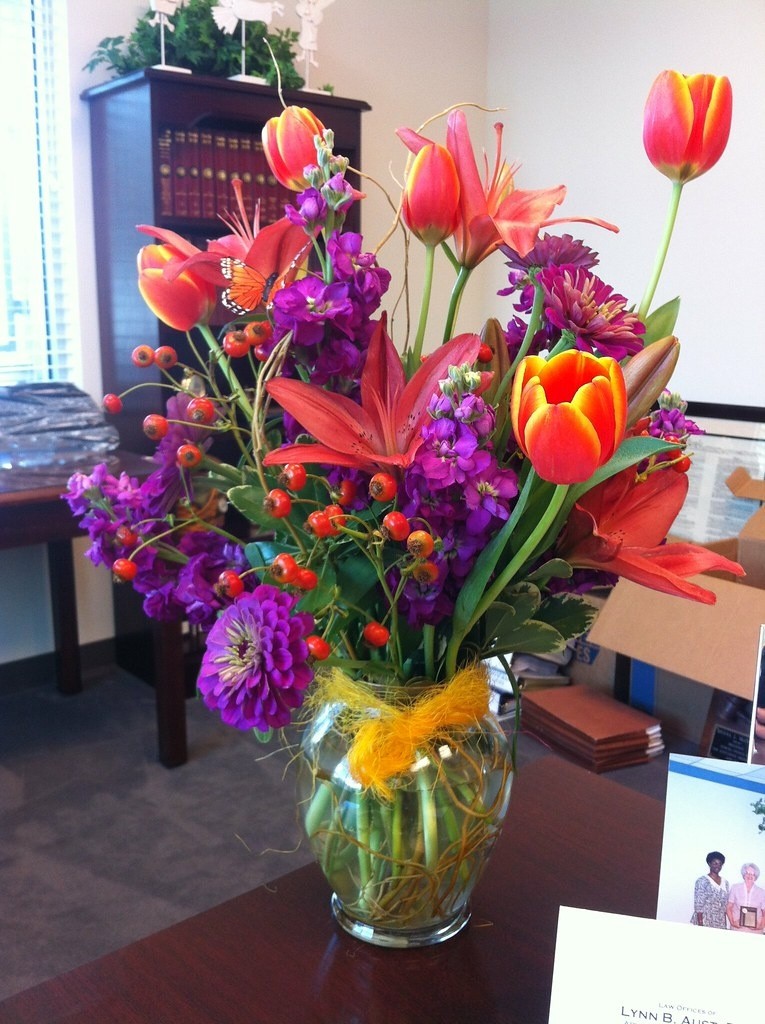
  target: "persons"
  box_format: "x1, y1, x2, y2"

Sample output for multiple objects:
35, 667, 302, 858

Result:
728, 863, 765, 934
690, 851, 730, 929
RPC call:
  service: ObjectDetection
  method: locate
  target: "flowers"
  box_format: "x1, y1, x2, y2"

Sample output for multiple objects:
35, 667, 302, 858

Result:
63, 64, 736, 926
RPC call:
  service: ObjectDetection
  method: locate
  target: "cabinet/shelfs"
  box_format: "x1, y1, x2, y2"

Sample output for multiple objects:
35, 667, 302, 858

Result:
81, 69, 371, 695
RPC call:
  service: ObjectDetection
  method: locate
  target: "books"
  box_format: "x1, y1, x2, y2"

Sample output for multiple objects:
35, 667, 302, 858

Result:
163, 233, 267, 326
481, 629, 575, 720
158, 122, 303, 226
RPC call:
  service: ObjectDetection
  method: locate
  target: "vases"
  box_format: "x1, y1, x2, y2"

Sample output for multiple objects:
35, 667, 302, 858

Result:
294, 662, 514, 948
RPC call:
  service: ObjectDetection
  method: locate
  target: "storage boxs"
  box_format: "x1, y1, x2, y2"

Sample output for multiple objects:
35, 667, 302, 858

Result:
552, 535, 697, 698
585, 465, 765, 766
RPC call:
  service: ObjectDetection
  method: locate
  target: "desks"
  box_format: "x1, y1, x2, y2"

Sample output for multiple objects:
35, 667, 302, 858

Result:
0, 754, 665, 1024
0, 446, 194, 765
